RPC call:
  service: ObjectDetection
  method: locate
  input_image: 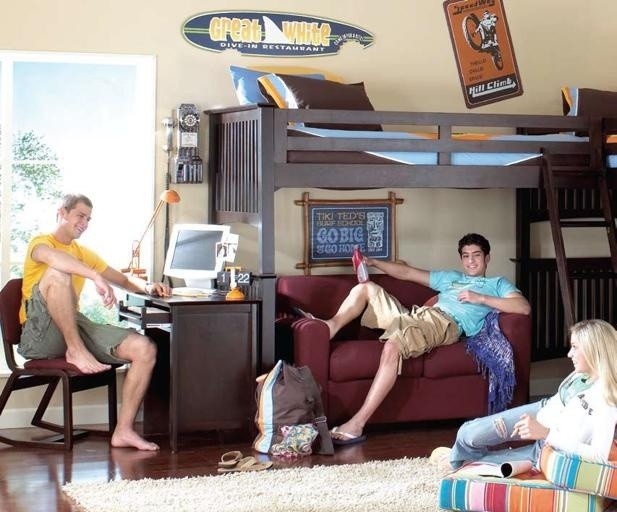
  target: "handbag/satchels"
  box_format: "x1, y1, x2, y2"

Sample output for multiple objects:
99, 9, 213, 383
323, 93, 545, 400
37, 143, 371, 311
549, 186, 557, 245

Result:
253, 360, 322, 453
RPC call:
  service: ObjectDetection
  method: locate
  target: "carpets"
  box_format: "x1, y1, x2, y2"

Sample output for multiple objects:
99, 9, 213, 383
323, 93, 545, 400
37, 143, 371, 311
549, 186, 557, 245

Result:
58, 458, 471, 512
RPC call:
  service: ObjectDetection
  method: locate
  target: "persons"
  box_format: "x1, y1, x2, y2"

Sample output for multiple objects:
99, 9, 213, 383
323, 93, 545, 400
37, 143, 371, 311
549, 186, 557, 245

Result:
429, 318, 617, 472
471, 10, 498, 47
16, 192, 171, 451
293, 230, 533, 447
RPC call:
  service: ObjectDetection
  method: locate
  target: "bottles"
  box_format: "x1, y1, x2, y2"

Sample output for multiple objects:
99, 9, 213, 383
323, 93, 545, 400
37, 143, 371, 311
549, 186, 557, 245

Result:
352, 245, 370, 284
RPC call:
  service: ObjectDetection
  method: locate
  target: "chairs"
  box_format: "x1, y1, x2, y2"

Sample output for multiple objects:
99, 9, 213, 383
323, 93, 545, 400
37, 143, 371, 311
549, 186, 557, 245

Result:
0, 279, 122, 450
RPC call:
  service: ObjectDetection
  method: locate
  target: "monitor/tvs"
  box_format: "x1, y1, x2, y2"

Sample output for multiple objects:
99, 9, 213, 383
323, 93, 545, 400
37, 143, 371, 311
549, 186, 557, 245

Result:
163, 224, 231, 298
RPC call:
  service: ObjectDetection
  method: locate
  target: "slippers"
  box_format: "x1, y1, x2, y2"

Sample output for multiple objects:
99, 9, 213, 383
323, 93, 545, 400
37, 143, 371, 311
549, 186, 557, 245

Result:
327, 426, 366, 445
294, 307, 315, 320
218, 451, 273, 474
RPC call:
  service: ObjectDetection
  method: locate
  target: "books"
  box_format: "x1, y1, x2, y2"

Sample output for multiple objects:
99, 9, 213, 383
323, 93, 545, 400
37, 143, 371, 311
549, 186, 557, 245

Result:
472, 460, 532, 479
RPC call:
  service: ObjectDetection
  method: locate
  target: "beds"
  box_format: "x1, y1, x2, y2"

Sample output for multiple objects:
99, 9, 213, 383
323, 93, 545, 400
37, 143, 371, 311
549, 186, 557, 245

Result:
197, 103, 616, 375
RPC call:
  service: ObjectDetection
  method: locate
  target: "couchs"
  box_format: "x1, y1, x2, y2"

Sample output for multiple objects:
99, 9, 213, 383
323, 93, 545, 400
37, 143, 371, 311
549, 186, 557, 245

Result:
276, 274, 531, 426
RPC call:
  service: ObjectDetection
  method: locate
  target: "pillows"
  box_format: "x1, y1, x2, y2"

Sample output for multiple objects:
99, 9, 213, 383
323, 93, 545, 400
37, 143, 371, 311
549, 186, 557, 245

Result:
537, 440, 617, 501
258, 74, 384, 133
439, 451, 615, 512
230, 65, 344, 107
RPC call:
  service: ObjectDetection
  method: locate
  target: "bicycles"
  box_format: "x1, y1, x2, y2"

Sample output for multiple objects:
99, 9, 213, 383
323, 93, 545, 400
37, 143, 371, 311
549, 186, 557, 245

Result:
463, 12, 502, 69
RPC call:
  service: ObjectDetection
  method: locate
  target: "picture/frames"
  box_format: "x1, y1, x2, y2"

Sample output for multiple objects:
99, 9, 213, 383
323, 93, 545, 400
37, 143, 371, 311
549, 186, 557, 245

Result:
294, 191, 403, 276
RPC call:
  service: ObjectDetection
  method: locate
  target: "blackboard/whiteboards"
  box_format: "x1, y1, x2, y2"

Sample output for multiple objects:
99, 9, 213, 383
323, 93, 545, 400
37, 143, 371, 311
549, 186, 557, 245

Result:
304, 199, 396, 267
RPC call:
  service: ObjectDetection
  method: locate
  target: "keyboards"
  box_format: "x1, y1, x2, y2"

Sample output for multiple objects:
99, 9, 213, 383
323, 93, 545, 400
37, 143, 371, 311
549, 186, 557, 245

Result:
127, 305, 169, 315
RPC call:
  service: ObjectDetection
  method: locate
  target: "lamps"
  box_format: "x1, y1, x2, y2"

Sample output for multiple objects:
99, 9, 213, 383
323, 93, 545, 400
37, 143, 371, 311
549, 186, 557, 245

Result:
122, 189, 180, 281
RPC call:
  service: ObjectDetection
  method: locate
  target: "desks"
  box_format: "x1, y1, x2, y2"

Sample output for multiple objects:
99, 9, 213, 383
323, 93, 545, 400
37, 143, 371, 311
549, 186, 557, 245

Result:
108, 284, 263, 451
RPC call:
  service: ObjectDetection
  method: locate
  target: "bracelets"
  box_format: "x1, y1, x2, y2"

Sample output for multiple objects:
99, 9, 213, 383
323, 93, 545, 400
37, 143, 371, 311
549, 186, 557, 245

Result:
145, 282, 154, 294
